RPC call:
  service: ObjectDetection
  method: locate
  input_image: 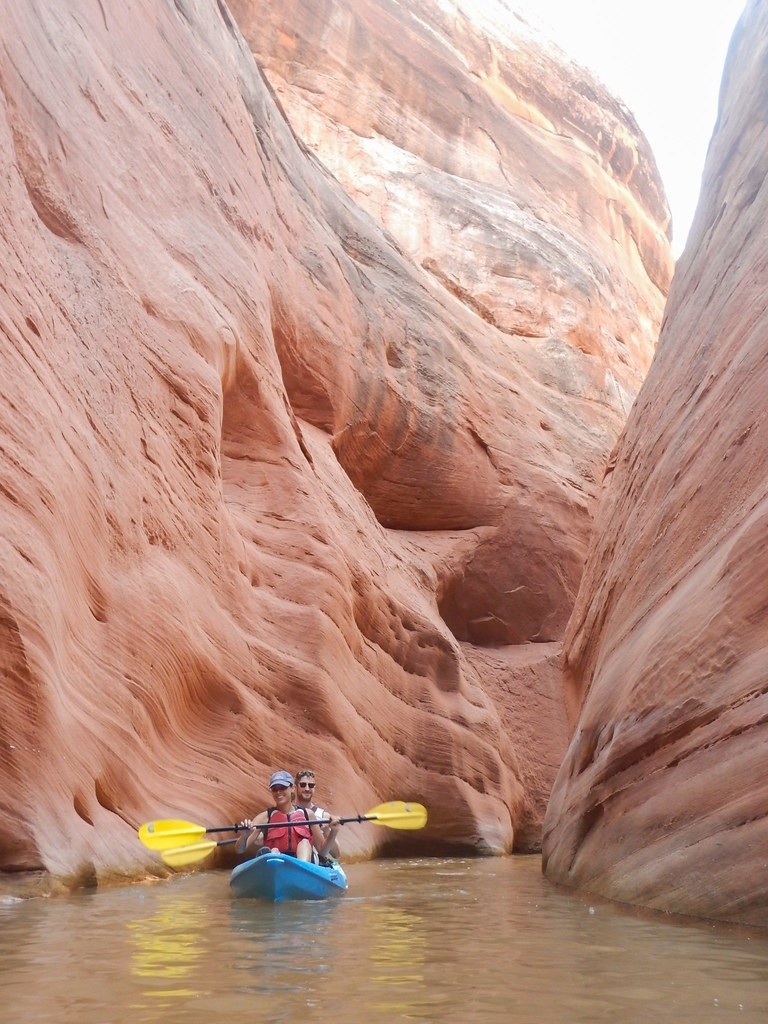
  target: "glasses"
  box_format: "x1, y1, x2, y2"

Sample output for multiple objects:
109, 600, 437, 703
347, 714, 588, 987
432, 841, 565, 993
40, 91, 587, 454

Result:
297, 782, 316, 788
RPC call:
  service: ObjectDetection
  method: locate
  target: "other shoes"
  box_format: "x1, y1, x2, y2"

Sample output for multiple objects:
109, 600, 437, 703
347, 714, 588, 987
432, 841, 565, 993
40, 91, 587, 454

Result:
256, 847, 272, 858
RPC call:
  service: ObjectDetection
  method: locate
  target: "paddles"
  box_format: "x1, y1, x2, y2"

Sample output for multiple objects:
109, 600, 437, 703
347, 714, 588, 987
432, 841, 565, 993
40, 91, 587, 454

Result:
138, 802, 427, 850
160, 800, 405, 867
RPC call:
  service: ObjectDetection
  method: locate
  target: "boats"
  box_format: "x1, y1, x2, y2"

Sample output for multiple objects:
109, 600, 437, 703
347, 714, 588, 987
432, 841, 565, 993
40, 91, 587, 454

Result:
230, 848, 348, 903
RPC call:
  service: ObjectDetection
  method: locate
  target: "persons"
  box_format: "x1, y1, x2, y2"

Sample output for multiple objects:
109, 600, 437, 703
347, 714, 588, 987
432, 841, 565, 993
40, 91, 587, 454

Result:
234, 769, 341, 861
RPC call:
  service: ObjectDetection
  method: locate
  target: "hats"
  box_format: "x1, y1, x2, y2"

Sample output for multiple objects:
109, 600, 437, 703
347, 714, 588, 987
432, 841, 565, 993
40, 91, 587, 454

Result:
269, 770, 294, 789
